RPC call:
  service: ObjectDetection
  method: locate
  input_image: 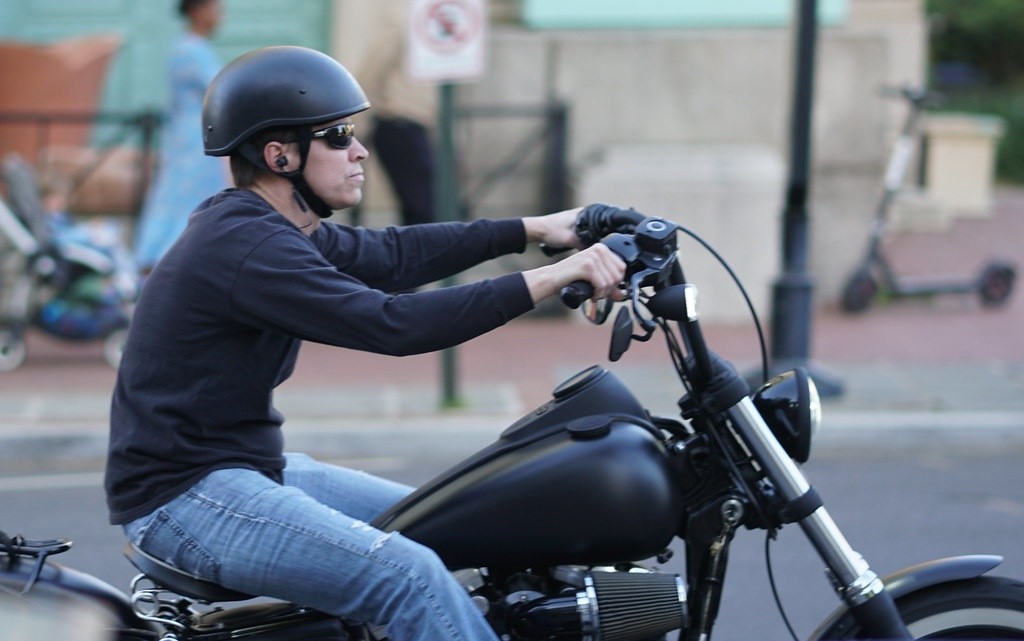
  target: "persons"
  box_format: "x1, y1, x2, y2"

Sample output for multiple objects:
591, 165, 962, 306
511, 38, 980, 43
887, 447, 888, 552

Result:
42, 189, 139, 299
132, 0, 227, 278
104, 46, 628, 641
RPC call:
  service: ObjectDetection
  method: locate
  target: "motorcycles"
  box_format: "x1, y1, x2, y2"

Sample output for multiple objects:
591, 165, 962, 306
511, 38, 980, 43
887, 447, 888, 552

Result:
0, 202, 1022, 640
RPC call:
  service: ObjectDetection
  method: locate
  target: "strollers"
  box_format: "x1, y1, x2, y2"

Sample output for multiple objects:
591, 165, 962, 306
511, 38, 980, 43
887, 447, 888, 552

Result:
0, 154, 144, 369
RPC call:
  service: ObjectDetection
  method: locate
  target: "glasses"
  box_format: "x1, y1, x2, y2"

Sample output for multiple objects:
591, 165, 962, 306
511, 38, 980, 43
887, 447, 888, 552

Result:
289, 122, 356, 151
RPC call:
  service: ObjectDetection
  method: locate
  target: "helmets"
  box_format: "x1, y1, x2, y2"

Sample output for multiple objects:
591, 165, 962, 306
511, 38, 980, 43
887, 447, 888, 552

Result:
201, 46, 372, 156
